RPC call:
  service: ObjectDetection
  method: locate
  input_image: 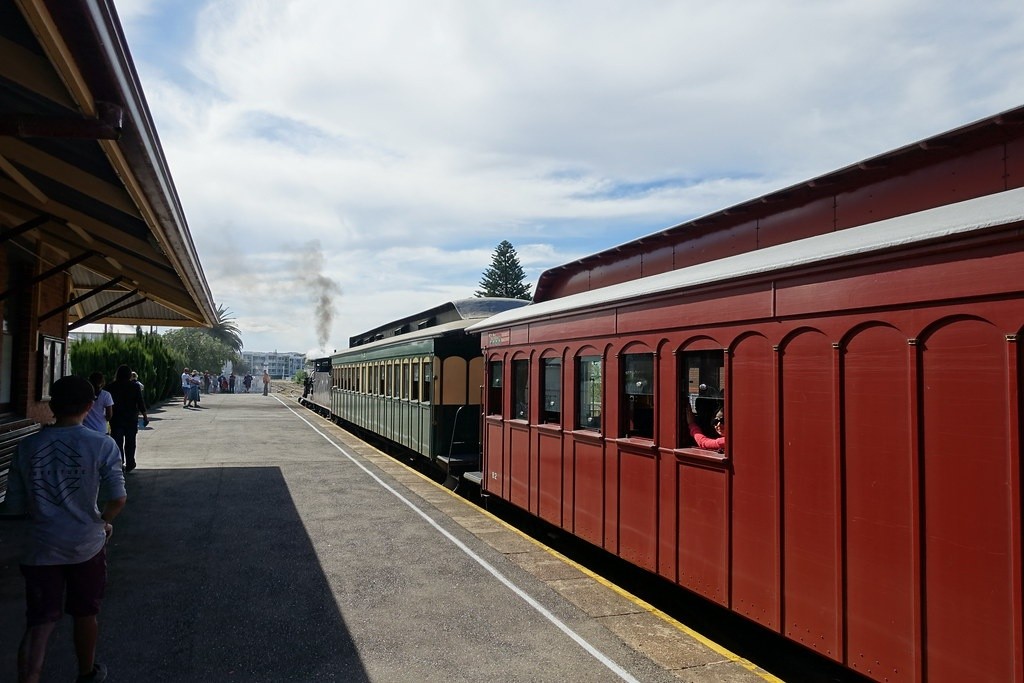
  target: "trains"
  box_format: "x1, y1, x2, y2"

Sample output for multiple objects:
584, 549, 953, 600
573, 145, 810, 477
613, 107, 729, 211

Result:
297, 104, 1024, 680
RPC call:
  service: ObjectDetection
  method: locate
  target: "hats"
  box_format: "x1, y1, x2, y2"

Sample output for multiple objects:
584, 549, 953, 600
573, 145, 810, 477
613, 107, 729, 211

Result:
190, 370, 199, 375
49, 375, 95, 405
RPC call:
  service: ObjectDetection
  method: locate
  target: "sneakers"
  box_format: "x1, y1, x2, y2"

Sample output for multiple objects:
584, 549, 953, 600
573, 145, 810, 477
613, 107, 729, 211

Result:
90, 663, 107, 683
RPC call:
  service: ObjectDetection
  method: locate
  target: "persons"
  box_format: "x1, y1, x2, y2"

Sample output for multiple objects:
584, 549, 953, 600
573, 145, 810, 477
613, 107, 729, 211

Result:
628, 377, 653, 439
182, 368, 235, 408
83, 364, 149, 473
686, 384, 724, 454
263, 370, 270, 396
5, 376, 128, 683
303, 373, 312, 398
243, 371, 253, 393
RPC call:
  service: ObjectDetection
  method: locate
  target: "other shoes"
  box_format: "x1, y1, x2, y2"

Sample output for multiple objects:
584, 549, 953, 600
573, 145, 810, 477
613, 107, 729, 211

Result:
187, 404, 194, 407
194, 405, 200, 408
125, 462, 136, 473
